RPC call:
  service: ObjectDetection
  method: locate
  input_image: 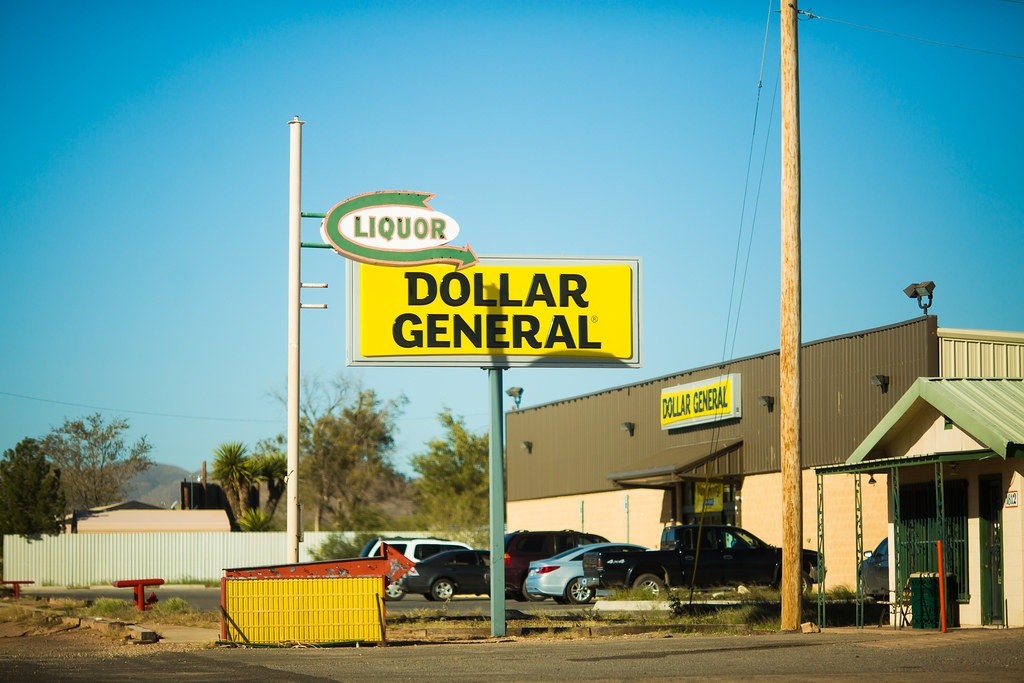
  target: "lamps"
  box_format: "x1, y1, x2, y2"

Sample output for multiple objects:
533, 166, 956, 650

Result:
757, 395, 775, 406
867, 473, 877, 484
621, 422, 635, 431
903, 280, 936, 313
870, 375, 890, 386
947, 462, 962, 477
506, 386, 524, 408
521, 441, 531, 449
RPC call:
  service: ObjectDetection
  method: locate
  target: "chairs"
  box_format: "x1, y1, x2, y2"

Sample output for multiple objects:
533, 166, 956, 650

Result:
877, 578, 912, 627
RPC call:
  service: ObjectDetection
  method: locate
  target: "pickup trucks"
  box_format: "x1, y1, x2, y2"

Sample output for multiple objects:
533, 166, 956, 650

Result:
577, 524, 827, 597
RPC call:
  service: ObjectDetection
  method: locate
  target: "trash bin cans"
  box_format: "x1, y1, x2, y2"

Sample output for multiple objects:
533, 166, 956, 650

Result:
909, 571, 959, 629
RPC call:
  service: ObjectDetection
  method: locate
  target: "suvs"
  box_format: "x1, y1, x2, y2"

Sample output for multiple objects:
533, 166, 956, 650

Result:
484, 530, 610, 601
360, 537, 474, 601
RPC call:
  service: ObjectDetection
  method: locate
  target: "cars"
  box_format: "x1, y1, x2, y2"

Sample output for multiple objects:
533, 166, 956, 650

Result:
858, 537, 889, 598
526, 542, 652, 604
400, 549, 490, 601
